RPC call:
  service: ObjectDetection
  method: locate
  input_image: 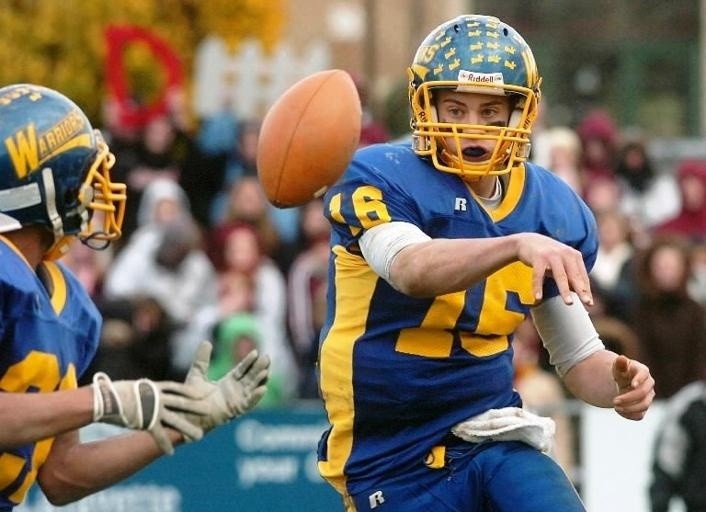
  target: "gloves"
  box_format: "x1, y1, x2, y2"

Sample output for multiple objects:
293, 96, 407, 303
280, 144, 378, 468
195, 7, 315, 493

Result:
176, 342, 269, 443
95, 372, 209, 455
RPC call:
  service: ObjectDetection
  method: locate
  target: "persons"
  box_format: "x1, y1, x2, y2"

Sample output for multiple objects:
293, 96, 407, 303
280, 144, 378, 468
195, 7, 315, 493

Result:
0, 82, 271, 511
316, 14, 656, 510
512, 114, 705, 511
56, 67, 388, 404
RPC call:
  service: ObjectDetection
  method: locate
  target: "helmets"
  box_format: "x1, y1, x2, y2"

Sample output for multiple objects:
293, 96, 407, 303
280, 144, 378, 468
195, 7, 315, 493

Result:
406, 15, 543, 175
0, 84, 126, 240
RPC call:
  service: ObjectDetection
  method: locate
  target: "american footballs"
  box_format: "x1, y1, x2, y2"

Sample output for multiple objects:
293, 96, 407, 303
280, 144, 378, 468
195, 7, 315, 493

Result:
256, 70, 362, 209
